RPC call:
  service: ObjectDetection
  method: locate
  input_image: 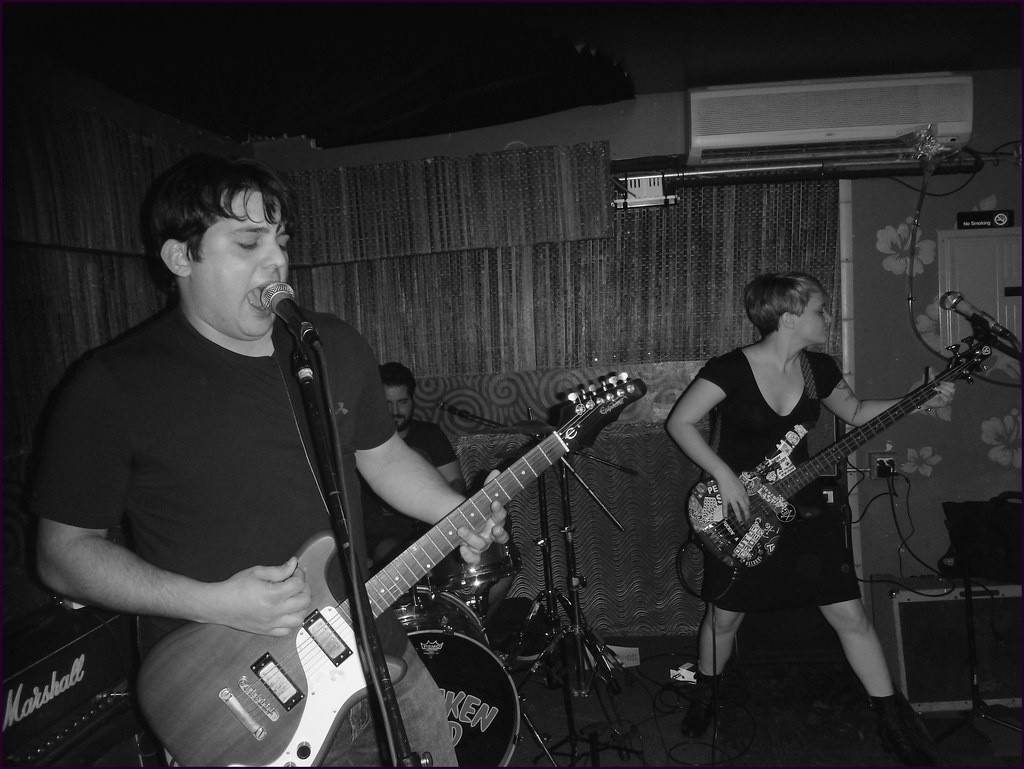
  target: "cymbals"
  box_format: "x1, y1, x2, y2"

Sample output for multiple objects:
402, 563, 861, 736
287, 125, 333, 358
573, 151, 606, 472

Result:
468, 419, 556, 435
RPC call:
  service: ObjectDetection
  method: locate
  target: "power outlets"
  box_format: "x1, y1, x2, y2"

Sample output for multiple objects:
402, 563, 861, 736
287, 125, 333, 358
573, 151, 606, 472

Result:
868, 452, 898, 480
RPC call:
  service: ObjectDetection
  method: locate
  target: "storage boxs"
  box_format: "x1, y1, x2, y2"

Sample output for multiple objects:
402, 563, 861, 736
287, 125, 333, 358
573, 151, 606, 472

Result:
870, 572, 1021, 715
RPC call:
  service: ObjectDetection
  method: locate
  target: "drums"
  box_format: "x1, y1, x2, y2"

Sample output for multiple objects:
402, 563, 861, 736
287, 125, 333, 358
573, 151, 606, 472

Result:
388, 585, 521, 769
435, 522, 523, 590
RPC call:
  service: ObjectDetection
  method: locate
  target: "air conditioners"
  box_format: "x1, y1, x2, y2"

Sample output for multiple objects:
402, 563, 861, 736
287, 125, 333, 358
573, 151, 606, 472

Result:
686, 71, 973, 167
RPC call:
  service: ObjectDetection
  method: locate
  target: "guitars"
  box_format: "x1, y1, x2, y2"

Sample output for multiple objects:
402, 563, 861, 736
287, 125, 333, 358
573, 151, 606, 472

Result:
135, 371, 648, 769
686, 336, 996, 572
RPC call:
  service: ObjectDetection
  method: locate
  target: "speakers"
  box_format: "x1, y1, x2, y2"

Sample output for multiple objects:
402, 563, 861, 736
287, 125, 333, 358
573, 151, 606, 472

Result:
29, 694, 168, 767
889, 572, 1024, 713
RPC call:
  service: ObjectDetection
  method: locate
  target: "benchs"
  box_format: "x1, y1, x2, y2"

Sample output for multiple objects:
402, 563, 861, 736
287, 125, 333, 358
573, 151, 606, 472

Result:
413, 416, 723, 635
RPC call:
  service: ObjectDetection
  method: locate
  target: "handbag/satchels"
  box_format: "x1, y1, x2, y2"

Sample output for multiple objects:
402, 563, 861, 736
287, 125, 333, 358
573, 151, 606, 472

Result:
937, 491, 1023, 585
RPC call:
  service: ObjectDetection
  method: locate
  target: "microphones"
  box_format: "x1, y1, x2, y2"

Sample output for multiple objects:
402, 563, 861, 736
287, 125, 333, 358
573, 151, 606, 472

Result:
939, 290, 1014, 340
435, 397, 471, 418
260, 280, 320, 346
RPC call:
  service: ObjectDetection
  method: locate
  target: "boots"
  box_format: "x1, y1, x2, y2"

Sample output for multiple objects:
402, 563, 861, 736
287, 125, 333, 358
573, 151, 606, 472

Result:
683, 660, 723, 736
868, 692, 925, 768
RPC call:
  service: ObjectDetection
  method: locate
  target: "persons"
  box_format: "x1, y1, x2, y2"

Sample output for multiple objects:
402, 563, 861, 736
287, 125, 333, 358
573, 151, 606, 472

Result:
352, 361, 517, 622
663, 270, 956, 768
27, 151, 510, 769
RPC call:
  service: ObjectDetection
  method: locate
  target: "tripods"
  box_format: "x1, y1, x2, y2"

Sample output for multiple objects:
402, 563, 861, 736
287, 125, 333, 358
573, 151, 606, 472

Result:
936, 557, 1024, 745
461, 412, 642, 767
